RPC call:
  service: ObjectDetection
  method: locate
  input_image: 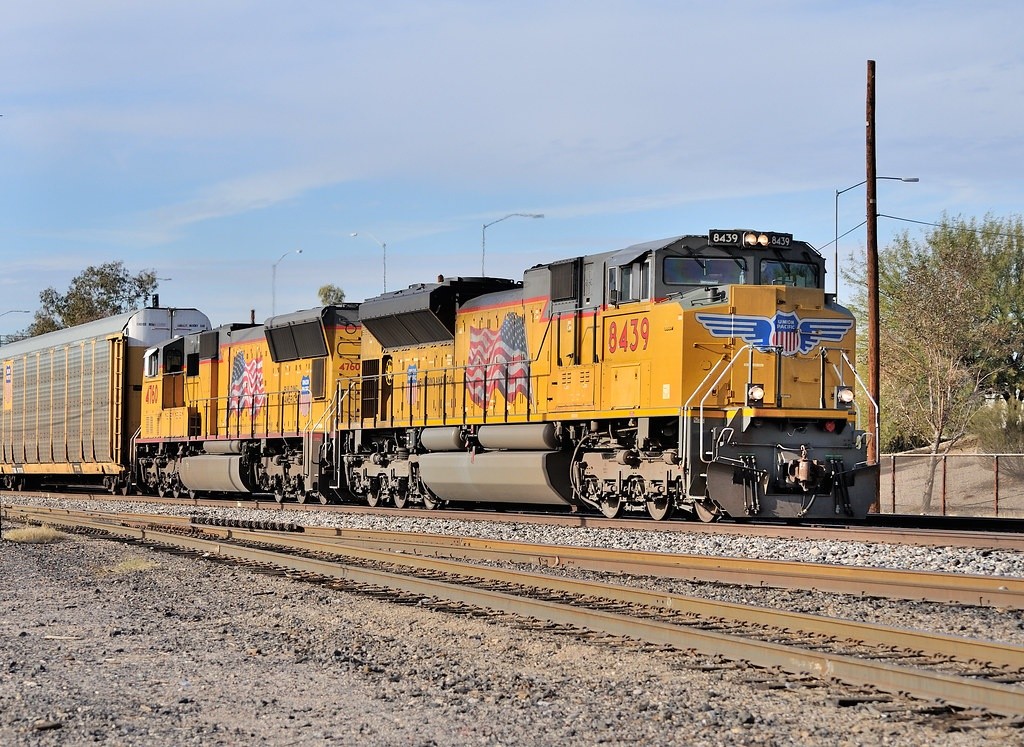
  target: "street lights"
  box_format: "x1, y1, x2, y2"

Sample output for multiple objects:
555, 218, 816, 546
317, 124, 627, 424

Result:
834, 177, 919, 307
482, 212, 545, 278
351, 231, 387, 295
271, 249, 303, 318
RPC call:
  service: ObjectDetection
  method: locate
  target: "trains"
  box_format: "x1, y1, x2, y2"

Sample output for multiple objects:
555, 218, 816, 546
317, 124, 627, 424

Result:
0, 230, 882, 527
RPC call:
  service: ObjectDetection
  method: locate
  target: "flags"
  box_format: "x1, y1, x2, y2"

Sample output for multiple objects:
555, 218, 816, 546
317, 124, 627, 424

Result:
464, 313, 534, 412
228, 352, 267, 420
